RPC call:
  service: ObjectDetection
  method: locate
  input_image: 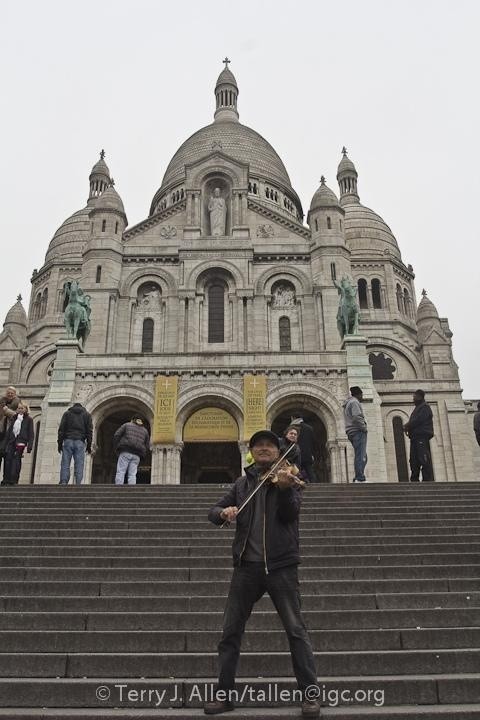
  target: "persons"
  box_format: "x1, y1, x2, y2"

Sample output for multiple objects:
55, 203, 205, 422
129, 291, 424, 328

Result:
342, 386, 369, 483
206, 428, 320, 700
473, 402, 480, 446
113, 412, 149, 485
278, 426, 302, 473
208, 187, 227, 236
403, 389, 435, 482
58, 403, 93, 485
0, 386, 34, 484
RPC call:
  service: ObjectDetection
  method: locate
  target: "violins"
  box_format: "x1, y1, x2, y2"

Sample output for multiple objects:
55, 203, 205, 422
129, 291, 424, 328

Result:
259, 455, 310, 492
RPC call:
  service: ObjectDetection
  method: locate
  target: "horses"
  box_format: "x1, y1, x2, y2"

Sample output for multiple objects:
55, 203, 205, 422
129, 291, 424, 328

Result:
63, 279, 93, 349
335, 275, 361, 340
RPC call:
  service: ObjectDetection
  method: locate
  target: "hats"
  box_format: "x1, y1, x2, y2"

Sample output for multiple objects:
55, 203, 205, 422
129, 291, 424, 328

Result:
249, 429, 281, 452
414, 388, 426, 398
349, 386, 364, 396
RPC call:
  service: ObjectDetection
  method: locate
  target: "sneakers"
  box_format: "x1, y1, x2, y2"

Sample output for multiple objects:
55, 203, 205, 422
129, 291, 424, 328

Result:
301, 694, 322, 717
203, 697, 235, 713
